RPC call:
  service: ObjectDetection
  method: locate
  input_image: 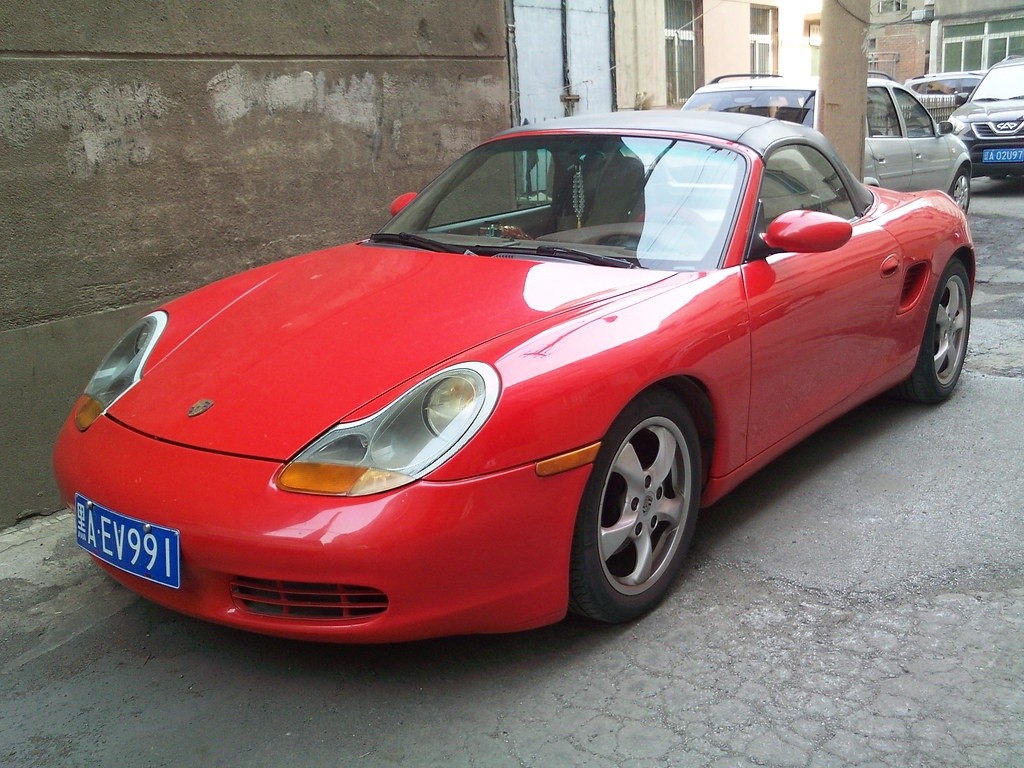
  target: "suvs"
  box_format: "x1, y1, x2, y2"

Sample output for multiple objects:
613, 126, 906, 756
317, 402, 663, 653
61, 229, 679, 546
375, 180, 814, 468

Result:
947, 54, 1024, 181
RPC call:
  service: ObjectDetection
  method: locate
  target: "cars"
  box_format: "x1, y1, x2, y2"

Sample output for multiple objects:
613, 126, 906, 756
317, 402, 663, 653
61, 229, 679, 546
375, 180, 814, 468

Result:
639, 73, 972, 218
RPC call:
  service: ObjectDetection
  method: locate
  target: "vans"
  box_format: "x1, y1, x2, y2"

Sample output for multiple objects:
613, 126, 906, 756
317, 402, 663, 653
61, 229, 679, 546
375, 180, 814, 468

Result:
903, 71, 987, 123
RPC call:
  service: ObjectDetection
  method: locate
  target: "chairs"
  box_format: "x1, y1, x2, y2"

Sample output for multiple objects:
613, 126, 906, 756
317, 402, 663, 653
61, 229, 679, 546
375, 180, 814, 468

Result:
544, 156, 644, 235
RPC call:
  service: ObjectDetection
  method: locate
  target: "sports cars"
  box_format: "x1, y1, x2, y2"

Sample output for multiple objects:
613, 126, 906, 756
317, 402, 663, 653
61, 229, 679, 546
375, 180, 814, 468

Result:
51, 110, 978, 646
